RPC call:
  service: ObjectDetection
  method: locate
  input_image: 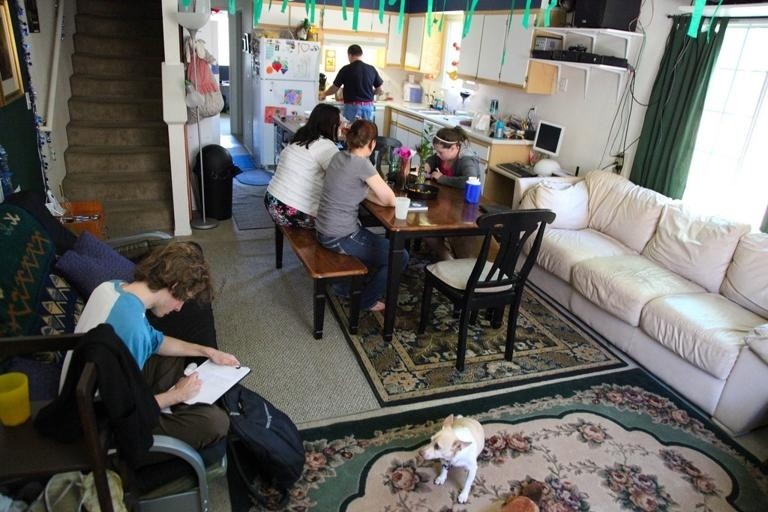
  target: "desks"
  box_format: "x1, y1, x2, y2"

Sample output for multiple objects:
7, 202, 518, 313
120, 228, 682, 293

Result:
0, 330, 143, 512
366, 172, 511, 343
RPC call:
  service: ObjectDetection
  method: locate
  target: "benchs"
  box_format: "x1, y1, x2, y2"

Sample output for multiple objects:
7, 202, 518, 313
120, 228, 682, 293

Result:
448, 232, 505, 324
273, 201, 366, 341
0, 199, 234, 511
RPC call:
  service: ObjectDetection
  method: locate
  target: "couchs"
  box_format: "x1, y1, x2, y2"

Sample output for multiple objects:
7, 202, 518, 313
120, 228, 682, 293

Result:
510, 171, 767, 441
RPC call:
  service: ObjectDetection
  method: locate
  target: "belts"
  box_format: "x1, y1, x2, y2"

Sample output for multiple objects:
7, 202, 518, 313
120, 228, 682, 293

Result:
344, 100, 373, 105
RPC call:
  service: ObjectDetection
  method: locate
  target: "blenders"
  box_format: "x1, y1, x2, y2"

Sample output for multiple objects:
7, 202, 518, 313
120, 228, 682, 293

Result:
402, 75, 422, 103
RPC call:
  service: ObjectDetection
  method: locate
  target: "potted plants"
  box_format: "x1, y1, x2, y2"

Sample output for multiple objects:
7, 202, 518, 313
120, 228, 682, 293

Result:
403, 122, 441, 201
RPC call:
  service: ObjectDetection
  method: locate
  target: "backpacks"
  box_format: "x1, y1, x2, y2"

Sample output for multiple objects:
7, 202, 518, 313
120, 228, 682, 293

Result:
216, 383, 305, 512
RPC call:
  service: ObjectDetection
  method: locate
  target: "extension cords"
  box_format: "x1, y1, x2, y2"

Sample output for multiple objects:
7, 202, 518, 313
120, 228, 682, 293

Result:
616, 152, 624, 173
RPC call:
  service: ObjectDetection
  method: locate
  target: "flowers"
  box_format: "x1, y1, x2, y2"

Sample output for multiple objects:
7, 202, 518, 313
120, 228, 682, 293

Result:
392, 146, 413, 181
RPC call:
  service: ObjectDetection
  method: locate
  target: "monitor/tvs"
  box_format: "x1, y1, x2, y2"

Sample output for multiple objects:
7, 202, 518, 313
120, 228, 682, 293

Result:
533, 120, 564, 157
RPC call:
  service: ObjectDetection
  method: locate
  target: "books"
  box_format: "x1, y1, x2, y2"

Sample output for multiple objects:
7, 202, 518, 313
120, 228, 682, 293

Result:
169, 359, 251, 405
409, 200, 428, 211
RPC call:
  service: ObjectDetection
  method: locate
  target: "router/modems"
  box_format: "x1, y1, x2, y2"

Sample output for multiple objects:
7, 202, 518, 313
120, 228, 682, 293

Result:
552, 165, 580, 177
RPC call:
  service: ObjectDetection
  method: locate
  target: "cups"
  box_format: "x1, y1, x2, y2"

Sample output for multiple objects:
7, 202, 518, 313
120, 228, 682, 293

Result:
464, 181, 481, 204
0, 371, 31, 427
394, 197, 411, 220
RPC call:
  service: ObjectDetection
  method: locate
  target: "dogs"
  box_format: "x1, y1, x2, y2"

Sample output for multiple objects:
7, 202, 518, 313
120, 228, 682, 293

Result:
419, 410, 486, 503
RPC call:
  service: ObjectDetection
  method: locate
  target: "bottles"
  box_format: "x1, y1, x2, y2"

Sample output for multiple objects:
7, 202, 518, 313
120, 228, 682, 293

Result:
494, 112, 504, 139
423, 91, 444, 112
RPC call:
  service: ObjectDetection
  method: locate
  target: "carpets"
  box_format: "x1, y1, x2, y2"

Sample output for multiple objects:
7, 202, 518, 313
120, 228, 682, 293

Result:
230, 153, 271, 186
237, 363, 766, 512
216, 144, 275, 230
316, 240, 628, 408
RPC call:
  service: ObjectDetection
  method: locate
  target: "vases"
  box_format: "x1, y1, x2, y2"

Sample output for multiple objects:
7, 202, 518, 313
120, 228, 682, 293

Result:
397, 162, 409, 191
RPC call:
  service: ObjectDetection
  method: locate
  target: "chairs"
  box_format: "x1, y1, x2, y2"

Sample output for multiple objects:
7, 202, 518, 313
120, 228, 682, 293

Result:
356, 136, 414, 255
418, 208, 559, 369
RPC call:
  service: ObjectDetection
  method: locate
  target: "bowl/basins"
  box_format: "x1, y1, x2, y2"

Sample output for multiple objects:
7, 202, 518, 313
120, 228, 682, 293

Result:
335, 88, 343, 101
411, 87, 422, 104
405, 183, 439, 200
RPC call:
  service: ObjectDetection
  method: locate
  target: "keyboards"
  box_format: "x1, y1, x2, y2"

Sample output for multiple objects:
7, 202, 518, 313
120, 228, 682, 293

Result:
497, 163, 536, 178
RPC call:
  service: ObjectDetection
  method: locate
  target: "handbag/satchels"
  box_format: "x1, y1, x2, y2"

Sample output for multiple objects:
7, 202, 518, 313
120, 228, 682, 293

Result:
185, 37, 225, 117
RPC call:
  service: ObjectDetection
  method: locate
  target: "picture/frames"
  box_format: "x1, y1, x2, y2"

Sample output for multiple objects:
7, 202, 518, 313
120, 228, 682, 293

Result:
0, 0, 25, 110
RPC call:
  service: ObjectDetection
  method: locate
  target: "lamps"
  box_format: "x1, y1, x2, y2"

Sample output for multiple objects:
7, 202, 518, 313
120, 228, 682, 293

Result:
171, 9, 221, 231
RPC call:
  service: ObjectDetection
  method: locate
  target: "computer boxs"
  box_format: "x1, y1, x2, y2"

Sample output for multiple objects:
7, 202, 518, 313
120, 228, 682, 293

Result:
574, 0, 642, 31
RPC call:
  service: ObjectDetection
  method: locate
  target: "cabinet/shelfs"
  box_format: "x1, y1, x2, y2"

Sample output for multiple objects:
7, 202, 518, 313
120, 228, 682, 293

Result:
389, 107, 424, 151
425, 121, 534, 206
456, 8, 485, 81
252, 0, 447, 80
337, 105, 388, 135
476, 6, 564, 96
56, 200, 107, 240
529, 24, 647, 101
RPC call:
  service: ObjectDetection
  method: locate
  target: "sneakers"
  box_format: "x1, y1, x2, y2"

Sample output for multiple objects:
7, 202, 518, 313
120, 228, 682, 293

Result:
370, 300, 386, 312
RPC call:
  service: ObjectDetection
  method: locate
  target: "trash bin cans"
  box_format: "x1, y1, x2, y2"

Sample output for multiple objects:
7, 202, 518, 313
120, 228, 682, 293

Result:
192, 144, 243, 221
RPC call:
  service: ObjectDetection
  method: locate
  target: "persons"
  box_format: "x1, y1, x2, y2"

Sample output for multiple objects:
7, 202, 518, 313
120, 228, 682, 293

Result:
264, 104, 340, 231
425, 126, 480, 259
58, 240, 239, 471
315, 119, 410, 311
319, 45, 384, 124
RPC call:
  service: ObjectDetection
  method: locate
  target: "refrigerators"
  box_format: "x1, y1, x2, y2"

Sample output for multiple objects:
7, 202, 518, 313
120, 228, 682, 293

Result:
252, 38, 319, 168
